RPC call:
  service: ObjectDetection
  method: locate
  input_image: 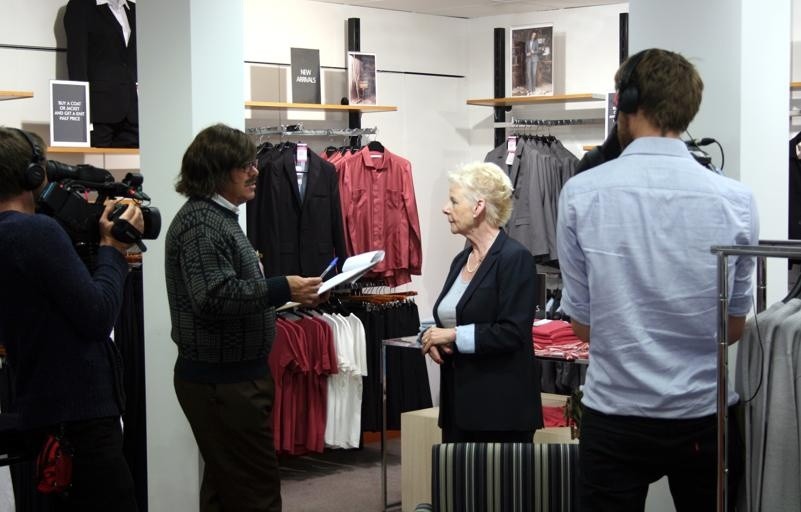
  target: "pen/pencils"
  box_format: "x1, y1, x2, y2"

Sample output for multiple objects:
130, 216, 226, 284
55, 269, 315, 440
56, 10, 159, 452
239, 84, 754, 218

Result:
321, 256, 339, 278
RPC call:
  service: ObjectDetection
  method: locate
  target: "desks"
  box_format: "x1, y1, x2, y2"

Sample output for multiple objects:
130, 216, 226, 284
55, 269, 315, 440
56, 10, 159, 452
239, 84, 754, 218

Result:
378, 328, 590, 511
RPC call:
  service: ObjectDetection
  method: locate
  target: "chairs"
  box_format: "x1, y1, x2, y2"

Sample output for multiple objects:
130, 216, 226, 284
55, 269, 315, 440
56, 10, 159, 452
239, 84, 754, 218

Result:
413, 439, 583, 511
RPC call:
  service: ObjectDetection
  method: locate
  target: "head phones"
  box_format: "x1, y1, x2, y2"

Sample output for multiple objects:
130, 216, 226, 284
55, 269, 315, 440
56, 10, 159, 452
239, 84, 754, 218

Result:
616, 49, 647, 115
6, 126, 46, 192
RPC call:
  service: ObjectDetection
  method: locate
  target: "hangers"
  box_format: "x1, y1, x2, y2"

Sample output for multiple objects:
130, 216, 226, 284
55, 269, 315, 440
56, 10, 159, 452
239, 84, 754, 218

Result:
507, 119, 559, 147
256, 121, 386, 174
272, 279, 419, 324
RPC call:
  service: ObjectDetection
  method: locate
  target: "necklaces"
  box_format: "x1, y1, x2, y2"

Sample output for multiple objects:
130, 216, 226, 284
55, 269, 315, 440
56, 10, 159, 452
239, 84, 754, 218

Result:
466, 251, 485, 273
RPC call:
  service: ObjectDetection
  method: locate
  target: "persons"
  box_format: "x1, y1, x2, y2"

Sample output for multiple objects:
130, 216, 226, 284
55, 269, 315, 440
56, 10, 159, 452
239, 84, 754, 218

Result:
554, 46, 761, 512
63, 0, 139, 149
524, 30, 539, 96
415, 161, 545, 444
